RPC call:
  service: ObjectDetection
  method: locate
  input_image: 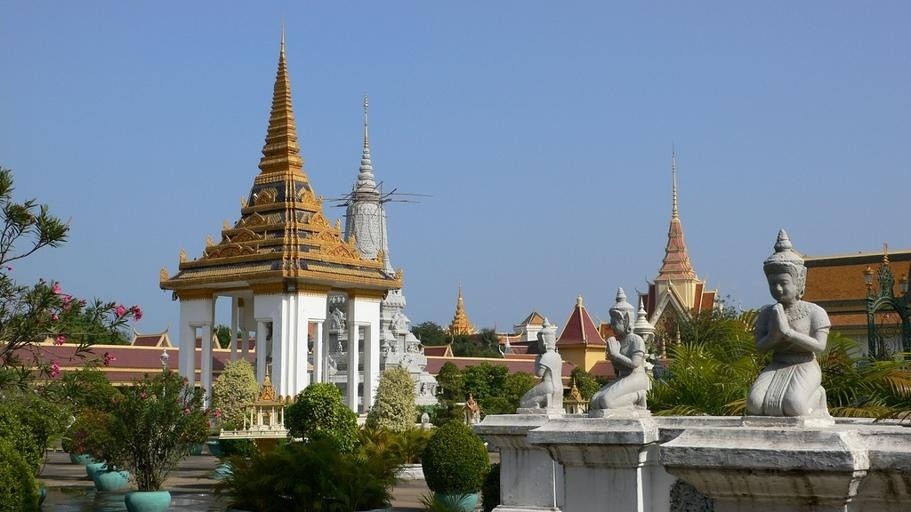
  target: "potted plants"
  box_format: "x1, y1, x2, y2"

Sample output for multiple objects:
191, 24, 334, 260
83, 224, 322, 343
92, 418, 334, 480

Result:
207, 439, 256, 480
38, 481, 49, 505
422, 417, 491, 512
62, 371, 212, 511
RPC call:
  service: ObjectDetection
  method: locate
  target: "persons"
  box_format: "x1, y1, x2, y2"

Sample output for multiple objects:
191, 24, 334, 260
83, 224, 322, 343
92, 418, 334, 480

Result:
591, 286, 650, 409
748, 229, 833, 416
462, 391, 479, 413
519, 316, 564, 408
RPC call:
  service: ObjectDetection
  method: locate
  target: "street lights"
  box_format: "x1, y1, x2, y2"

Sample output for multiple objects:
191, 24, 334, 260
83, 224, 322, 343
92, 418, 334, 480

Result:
160, 349, 170, 398
